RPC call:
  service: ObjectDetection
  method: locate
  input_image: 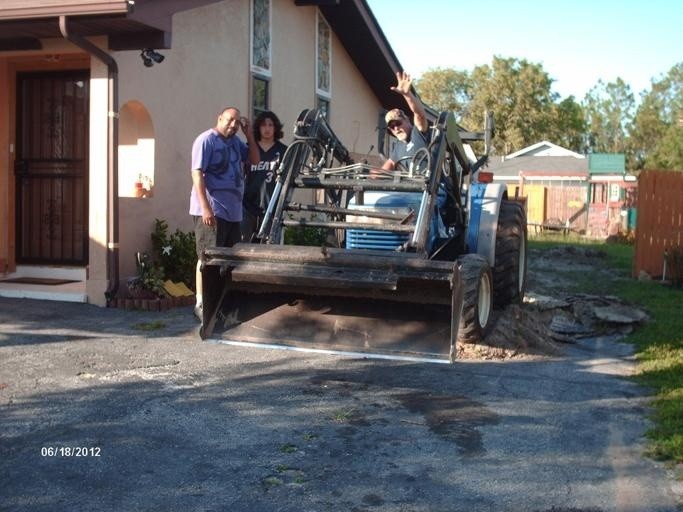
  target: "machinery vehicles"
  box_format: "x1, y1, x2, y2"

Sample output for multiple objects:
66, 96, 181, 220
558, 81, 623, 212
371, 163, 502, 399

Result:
196, 107, 526, 364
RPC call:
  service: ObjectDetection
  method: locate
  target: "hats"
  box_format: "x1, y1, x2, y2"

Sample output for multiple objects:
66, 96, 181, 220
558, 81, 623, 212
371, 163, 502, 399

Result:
384, 108, 404, 127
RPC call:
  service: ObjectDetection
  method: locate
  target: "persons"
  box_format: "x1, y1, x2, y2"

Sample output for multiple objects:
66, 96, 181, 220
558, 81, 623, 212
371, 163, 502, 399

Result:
187, 106, 259, 322
242, 110, 290, 243
368, 71, 451, 261
